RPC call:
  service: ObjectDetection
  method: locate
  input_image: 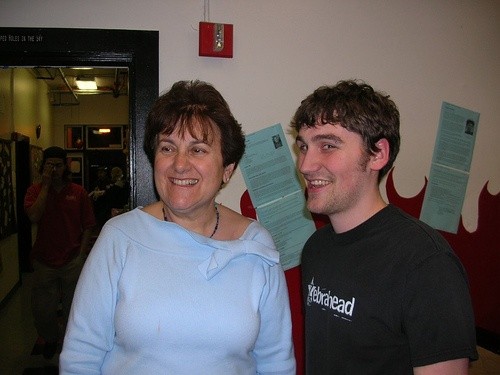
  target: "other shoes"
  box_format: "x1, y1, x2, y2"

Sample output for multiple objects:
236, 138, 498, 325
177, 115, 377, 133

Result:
42, 343, 58, 359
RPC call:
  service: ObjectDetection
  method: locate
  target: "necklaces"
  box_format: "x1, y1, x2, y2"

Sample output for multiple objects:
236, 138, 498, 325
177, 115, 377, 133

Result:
163, 201, 219, 238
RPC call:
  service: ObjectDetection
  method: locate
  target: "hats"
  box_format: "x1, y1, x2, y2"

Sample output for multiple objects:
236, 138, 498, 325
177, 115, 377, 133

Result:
42, 146, 67, 160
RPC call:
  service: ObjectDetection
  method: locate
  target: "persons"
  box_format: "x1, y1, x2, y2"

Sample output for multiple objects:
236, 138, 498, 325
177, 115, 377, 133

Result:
285, 78, 480, 375
87, 166, 129, 235
59, 78, 296, 375
24, 146, 96, 361
